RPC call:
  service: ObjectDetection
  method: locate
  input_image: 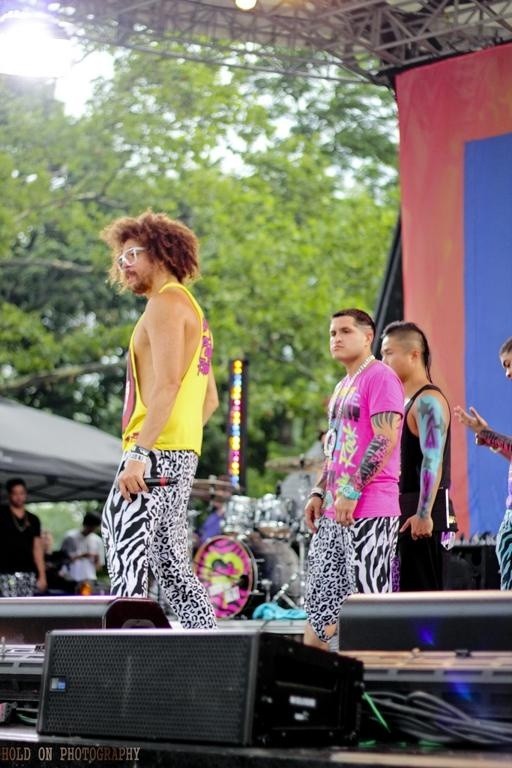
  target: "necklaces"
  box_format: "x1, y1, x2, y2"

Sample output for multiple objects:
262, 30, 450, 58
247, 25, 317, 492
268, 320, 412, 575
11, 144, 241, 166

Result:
324, 354, 375, 461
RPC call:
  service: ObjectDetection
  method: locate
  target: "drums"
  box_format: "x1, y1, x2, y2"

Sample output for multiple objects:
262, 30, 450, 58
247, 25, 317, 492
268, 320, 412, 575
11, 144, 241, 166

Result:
254, 493, 299, 539
194, 535, 300, 620
219, 495, 257, 536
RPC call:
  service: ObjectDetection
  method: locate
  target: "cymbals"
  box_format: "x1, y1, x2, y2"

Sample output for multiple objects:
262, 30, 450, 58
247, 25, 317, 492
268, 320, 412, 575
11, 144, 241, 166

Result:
190, 479, 240, 502
264, 456, 326, 471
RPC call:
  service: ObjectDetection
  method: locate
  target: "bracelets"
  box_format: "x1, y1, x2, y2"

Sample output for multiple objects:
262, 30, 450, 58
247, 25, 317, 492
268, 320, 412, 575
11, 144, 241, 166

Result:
311, 487, 324, 500
131, 445, 150, 457
128, 453, 146, 463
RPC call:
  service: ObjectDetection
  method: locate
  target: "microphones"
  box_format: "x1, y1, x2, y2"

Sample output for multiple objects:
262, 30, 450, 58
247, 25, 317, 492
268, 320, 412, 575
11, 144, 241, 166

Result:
144, 478, 179, 486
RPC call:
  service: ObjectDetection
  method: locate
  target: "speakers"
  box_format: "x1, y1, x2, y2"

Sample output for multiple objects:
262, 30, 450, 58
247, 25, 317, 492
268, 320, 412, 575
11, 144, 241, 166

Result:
35, 628, 365, 747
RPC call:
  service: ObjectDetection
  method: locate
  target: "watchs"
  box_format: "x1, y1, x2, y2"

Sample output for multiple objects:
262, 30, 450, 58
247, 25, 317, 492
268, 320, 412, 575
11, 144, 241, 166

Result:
342, 484, 362, 500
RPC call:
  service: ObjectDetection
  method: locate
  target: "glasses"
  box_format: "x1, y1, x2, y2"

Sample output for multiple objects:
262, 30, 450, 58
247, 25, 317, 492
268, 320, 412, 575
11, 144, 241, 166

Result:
117, 245, 146, 266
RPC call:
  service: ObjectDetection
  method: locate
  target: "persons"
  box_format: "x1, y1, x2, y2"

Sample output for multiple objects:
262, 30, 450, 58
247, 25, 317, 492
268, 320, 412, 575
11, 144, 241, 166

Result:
302, 309, 406, 651
453, 337, 512, 591
57, 512, 105, 595
0, 478, 48, 597
198, 505, 224, 545
100, 207, 219, 629
378, 320, 452, 591
